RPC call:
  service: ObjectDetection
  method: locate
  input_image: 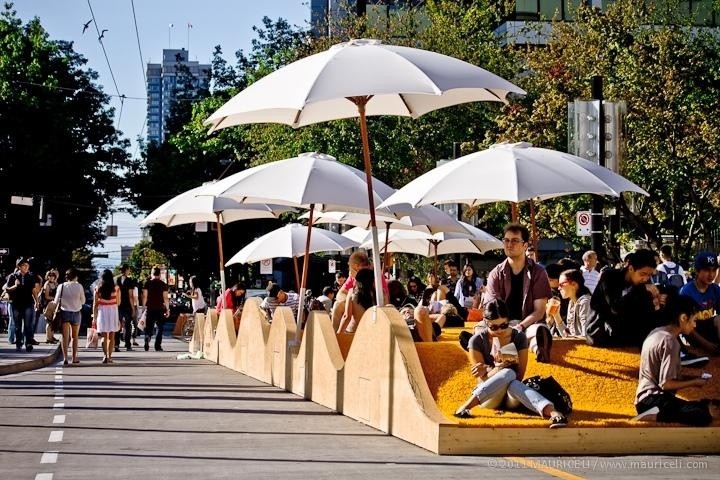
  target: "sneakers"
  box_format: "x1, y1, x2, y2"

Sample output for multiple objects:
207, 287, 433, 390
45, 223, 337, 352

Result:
680, 350, 710, 367
632, 406, 659, 420
536, 326, 552, 363
458, 331, 473, 352
10, 337, 163, 364
549, 417, 568, 428
454, 408, 470, 417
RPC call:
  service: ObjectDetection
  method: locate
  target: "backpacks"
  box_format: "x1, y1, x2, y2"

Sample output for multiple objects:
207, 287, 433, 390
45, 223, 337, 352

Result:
664, 264, 684, 287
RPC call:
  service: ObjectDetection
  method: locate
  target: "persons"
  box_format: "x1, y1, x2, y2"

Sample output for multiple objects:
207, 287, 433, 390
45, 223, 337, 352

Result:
453, 299, 570, 428
458, 223, 553, 363
0, 246, 714, 370
631, 295, 720, 427
195, 145, 504, 337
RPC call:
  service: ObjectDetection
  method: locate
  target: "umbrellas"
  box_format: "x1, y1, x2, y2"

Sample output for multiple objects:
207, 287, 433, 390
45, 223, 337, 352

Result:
204, 41, 526, 308
375, 142, 652, 264
137, 179, 300, 309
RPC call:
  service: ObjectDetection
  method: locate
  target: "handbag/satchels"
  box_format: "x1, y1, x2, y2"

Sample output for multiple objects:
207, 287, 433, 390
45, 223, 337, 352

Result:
522, 375, 572, 414
584, 310, 616, 347
37, 291, 47, 309
44, 301, 57, 320
86, 328, 98, 348
49, 312, 62, 333
2, 290, 9, 300
160, 307, 166, 324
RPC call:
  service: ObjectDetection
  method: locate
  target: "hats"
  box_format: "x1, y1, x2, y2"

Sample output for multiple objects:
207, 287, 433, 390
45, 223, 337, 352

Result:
266, 283, 281, 302
695, 252, 720, 268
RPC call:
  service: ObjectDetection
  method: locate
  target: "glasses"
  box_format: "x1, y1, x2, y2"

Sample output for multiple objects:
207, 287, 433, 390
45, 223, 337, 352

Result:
485, 321, 509, 331
559, 281, 568, 287
501, 237, 524, 246
